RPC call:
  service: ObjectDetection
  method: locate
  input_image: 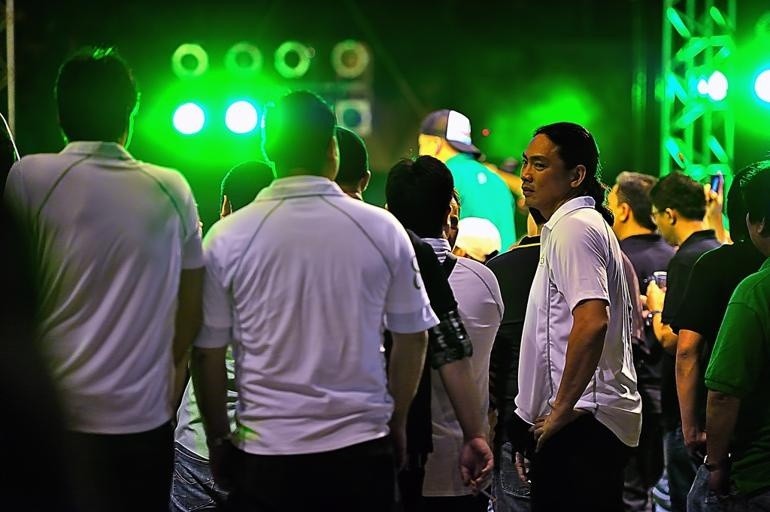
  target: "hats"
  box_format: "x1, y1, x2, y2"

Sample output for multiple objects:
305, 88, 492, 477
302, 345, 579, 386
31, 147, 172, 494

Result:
419, 107, 480, 154
455, 216, 502, 263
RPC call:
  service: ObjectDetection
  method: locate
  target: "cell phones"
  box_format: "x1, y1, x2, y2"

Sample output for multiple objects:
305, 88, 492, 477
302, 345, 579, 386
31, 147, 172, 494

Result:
709, 174, 720, 203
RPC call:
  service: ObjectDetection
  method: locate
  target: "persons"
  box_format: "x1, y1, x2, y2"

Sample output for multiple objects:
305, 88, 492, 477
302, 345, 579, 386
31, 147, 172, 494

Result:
608, 159, 770, 512
511, 122, 643, 512
170, 161, 275, 512
191, 90, 440, 512
328, 125, 549, 512
1, 54, 206, 510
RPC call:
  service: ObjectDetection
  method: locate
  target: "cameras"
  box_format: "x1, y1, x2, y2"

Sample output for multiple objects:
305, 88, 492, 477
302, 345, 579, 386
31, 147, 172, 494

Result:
654, 270, 667, 290
